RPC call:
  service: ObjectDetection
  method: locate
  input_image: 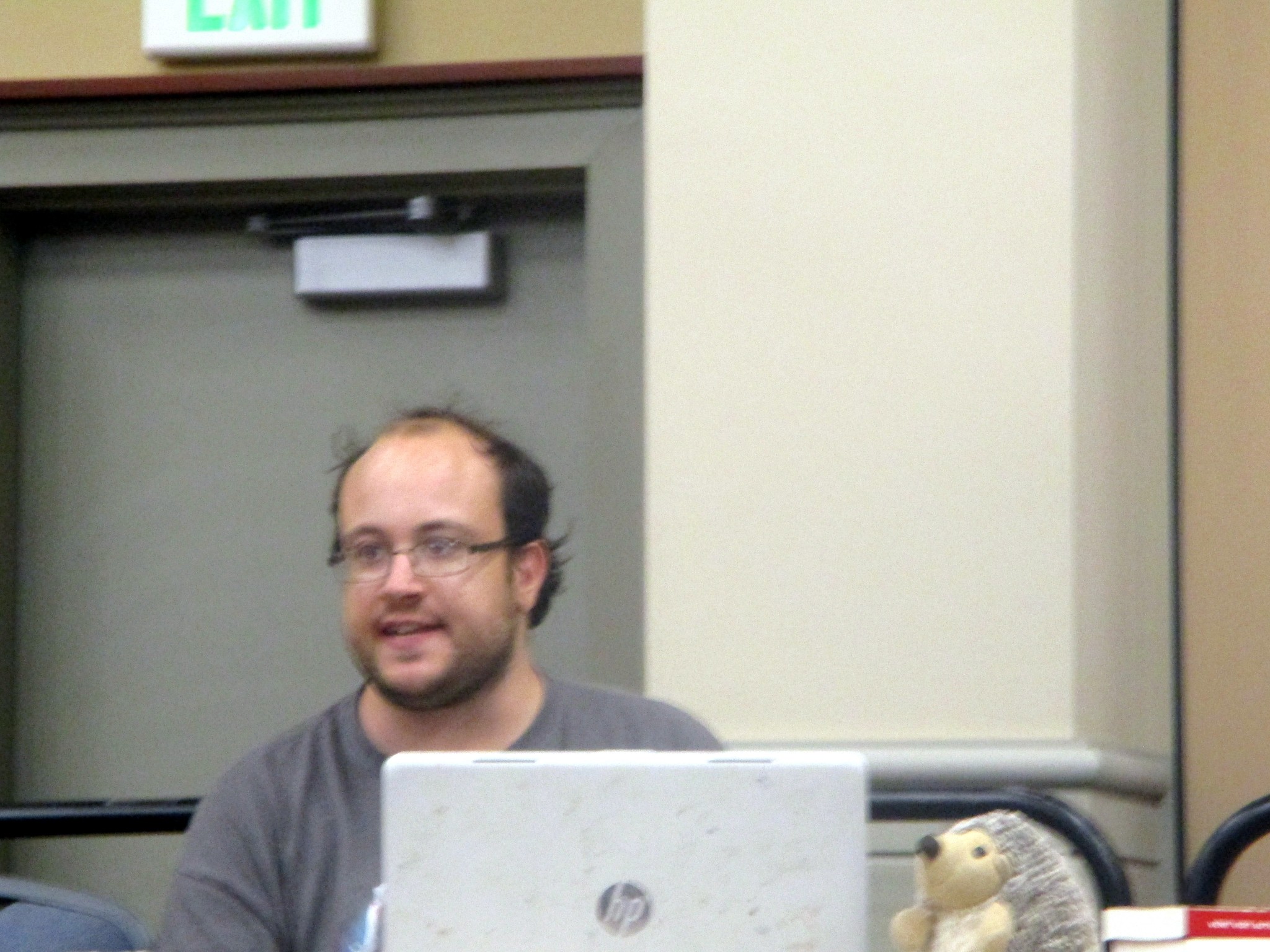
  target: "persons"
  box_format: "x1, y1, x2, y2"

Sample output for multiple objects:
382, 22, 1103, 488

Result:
148, 405, 721, 950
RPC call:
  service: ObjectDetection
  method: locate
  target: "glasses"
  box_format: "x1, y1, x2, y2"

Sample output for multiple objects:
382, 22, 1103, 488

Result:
330, 529, 519, 575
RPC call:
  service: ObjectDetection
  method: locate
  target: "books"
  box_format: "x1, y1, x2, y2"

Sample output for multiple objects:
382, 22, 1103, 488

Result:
1102, 905, 1270, 952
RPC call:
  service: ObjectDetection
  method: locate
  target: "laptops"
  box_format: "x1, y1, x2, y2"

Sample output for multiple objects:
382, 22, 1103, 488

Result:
378, 751, 872, 952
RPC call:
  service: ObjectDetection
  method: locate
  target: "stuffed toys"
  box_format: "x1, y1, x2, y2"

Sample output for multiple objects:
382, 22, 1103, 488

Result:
886, 806, 1102, 952
